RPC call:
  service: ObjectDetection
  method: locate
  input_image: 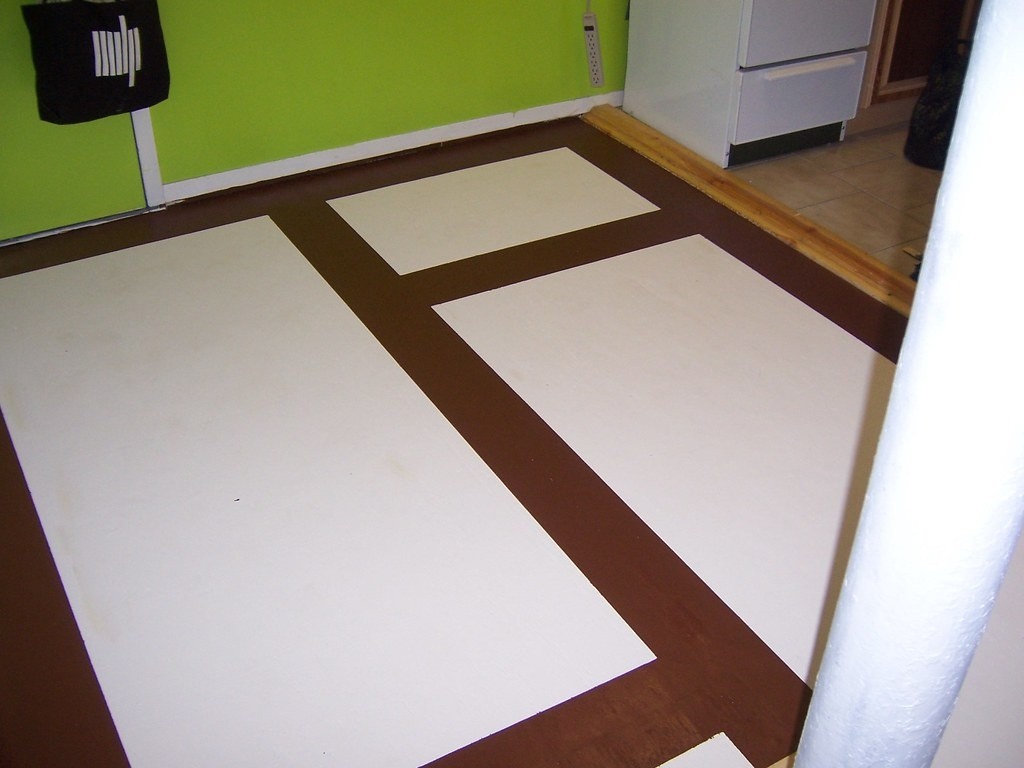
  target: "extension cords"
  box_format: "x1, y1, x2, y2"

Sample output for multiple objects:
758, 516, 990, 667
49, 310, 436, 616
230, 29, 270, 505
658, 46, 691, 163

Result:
583, 13, 605, 88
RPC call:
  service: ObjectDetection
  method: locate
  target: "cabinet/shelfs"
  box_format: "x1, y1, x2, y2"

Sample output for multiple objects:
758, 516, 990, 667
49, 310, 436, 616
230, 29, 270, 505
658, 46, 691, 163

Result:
624, 0, 879, 169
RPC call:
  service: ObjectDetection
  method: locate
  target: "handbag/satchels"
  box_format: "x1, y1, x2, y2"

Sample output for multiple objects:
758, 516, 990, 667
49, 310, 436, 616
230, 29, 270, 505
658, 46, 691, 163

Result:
21, 0, 170, 125
